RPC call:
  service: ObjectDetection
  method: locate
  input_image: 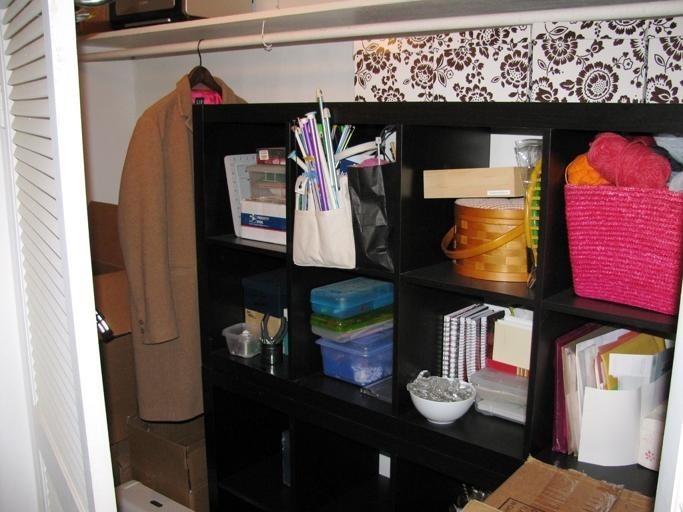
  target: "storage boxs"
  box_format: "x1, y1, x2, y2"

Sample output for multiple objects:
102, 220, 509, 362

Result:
310, 305, 395, 344
124, 410, 210, 512
238, 196, 287, 246
241, 275, 290, 324
314, 328, 394, 387
310, 277, 395, 320
457, 452, 655, 512
246, 164, 286, 204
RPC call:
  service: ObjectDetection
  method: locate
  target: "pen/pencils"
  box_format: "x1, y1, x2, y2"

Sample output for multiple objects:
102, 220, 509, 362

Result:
287, 88, 355, 211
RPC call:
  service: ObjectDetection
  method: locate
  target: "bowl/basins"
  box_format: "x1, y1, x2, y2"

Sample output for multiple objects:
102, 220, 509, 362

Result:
408, 377, 476, 425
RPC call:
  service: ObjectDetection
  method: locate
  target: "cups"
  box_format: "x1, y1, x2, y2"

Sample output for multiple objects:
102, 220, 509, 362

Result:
514, 140, 543, 198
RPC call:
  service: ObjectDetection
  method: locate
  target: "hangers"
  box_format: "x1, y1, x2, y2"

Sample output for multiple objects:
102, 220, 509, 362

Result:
186, 38, 222, 97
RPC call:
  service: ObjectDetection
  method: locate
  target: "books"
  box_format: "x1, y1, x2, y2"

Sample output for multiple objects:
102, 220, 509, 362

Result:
440, 302, 533, 384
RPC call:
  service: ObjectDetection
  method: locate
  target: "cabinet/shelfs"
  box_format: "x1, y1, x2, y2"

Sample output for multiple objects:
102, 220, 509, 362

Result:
190, 102, 683, 512
